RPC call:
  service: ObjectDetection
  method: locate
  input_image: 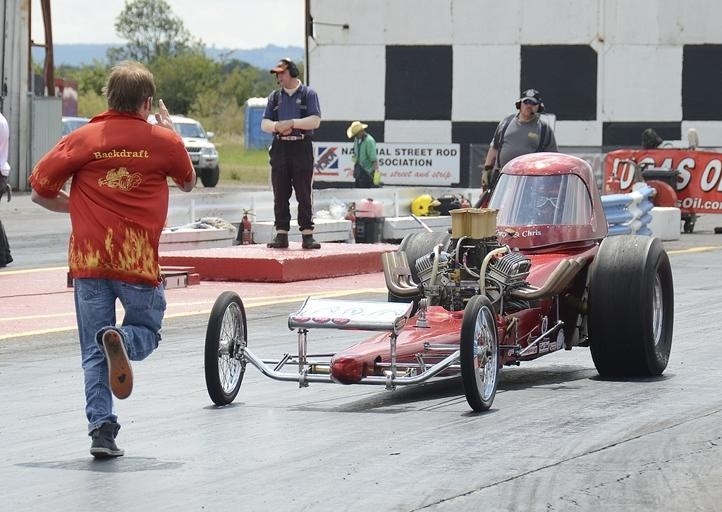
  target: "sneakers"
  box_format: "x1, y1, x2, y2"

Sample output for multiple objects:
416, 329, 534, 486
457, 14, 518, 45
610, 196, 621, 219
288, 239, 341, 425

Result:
102, 329, 133, 399
91, 422, 124, 457
267, 233, 288, 247
302, 234, 320, 249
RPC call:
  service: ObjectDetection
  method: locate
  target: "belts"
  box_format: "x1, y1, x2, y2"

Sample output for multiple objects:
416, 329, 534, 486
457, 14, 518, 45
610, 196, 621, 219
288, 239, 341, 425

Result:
277, 135, 305, 141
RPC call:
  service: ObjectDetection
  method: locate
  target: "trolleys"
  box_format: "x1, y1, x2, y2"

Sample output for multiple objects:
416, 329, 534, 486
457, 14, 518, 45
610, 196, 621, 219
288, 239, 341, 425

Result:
618, 158, 702, 233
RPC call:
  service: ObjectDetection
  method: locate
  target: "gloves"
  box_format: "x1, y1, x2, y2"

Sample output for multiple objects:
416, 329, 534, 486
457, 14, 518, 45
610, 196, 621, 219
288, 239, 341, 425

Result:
481, 169, 492, 187
275, 122, 293, 136
278, 120, 294, 133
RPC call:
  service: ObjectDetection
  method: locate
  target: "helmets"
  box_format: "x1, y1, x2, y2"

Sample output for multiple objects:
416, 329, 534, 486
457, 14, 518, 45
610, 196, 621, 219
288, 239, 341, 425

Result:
411, 195, 442, 216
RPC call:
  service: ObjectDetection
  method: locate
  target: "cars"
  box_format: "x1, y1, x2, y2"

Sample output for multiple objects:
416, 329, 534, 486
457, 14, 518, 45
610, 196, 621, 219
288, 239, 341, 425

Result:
58, 117, 92, 138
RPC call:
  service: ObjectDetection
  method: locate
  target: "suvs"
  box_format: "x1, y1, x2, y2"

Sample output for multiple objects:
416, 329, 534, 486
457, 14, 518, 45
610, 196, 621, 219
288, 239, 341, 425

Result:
145, 114, 220, 188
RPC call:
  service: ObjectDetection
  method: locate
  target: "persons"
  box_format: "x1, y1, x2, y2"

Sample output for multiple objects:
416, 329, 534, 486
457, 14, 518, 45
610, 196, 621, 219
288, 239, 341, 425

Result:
347, 121, 381, 188
528, 187, 561, 225
261, 58, 321, 248
481, 89, 558, 190
0, 112, 13, 268
27, 60, 197, 459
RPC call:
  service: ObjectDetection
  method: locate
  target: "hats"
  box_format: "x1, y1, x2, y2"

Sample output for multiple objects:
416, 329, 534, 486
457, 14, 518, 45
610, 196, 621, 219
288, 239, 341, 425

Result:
347, 122, 368, 139
270, 58, 292, 73
521, 89, 542, 104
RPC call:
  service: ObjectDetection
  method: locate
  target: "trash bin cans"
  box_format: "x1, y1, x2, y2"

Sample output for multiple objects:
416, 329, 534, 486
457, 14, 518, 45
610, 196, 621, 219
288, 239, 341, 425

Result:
356, 217, 384, 243
643, 167, 682, 189
